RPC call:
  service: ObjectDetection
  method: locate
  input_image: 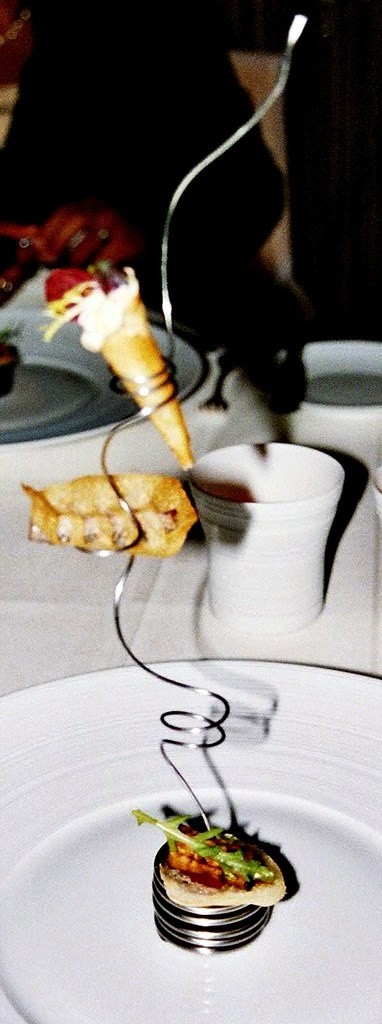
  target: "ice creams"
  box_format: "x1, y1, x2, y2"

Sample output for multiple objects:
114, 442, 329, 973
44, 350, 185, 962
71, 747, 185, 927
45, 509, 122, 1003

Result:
43, 266, 195, 471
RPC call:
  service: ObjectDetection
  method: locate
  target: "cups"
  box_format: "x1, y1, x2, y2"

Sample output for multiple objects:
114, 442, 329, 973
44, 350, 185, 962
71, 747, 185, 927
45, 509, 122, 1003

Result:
187, 443, 345, 638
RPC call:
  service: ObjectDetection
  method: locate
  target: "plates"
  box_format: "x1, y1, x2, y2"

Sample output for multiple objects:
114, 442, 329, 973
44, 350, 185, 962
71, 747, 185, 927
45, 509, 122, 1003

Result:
0, 306, 202, 449
296, 340, 382, 415
0, 656, 382, 1024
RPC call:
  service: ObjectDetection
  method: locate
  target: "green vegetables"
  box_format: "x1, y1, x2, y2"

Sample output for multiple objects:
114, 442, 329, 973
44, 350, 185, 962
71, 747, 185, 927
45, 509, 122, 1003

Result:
130, 808, 275, 884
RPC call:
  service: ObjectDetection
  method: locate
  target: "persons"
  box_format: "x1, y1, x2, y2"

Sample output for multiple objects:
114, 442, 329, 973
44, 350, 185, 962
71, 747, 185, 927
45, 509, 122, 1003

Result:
0, 0, 315, 356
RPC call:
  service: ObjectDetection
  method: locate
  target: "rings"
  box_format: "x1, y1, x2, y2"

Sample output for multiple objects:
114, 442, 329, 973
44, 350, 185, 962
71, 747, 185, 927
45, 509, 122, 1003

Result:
19, 238, 29, 248
88, 226, 109, 243
